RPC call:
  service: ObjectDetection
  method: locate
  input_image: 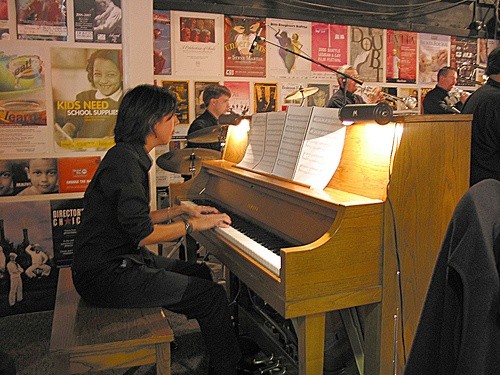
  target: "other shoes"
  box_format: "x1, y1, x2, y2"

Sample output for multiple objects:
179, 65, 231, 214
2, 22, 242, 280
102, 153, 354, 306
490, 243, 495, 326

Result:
237, 368, 262, 375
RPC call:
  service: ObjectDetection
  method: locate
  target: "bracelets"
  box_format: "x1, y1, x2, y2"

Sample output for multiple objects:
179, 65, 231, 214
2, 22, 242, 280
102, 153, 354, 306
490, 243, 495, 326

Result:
168, 206, 172, 223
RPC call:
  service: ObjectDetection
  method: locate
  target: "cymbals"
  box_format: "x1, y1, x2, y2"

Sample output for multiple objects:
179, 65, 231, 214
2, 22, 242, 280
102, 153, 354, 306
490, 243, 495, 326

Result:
285, 87, 320, 101
186, 124, 229, 143
156, 148, 223, 176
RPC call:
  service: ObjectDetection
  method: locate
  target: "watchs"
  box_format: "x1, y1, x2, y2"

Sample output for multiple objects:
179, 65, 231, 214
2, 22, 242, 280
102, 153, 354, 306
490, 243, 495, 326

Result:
183, 218, 193, 233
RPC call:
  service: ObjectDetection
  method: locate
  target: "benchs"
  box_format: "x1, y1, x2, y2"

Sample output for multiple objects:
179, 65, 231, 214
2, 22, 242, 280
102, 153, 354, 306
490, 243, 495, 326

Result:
48, 268, 175, 375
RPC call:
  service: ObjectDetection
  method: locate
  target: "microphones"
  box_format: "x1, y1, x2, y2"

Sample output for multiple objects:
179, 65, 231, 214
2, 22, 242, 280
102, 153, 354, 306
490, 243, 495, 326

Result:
249, 22, 265, 53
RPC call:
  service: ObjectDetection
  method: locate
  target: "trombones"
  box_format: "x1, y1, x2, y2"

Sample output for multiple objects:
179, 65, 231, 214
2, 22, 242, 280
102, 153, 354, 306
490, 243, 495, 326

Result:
457, 62, 488, 85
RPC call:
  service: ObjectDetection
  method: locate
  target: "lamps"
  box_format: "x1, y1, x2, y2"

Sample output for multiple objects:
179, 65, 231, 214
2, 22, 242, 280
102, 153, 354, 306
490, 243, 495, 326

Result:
217, 114, 251, 126
337, 102, 394, 126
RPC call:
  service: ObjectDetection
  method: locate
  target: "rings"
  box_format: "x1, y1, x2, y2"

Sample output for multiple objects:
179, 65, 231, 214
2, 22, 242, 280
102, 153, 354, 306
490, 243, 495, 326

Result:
201, 206, 203, 208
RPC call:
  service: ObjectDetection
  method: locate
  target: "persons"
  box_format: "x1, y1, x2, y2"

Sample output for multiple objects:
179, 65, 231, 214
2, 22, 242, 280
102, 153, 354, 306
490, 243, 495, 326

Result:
25, 243, 51, 279
180, 17, 215, 43
258, 92, 275, 112
0, 159, 59, 195
422, 67, 468, 115
56, 49, 123, 145
179, 83, 231, 261
19, 0, 66, 41
419, 52, 447, 82
83, 0, 122, 34
411, 90, 418, 107
460, 48, 500, 187
312, 85, 329, 106
71, 84, 265, 375
328, 64, 380, 108
7, 252, 24, 307
225, 16, 263, 55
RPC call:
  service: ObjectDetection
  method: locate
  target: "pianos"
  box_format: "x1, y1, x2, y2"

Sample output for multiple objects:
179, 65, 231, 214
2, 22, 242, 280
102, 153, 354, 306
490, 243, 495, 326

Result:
170, 113, 473, 375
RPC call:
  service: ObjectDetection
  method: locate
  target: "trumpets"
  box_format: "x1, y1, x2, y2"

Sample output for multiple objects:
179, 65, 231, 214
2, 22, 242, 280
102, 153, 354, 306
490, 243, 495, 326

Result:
356, 84, 417, 110
451, 85, 473, 100
